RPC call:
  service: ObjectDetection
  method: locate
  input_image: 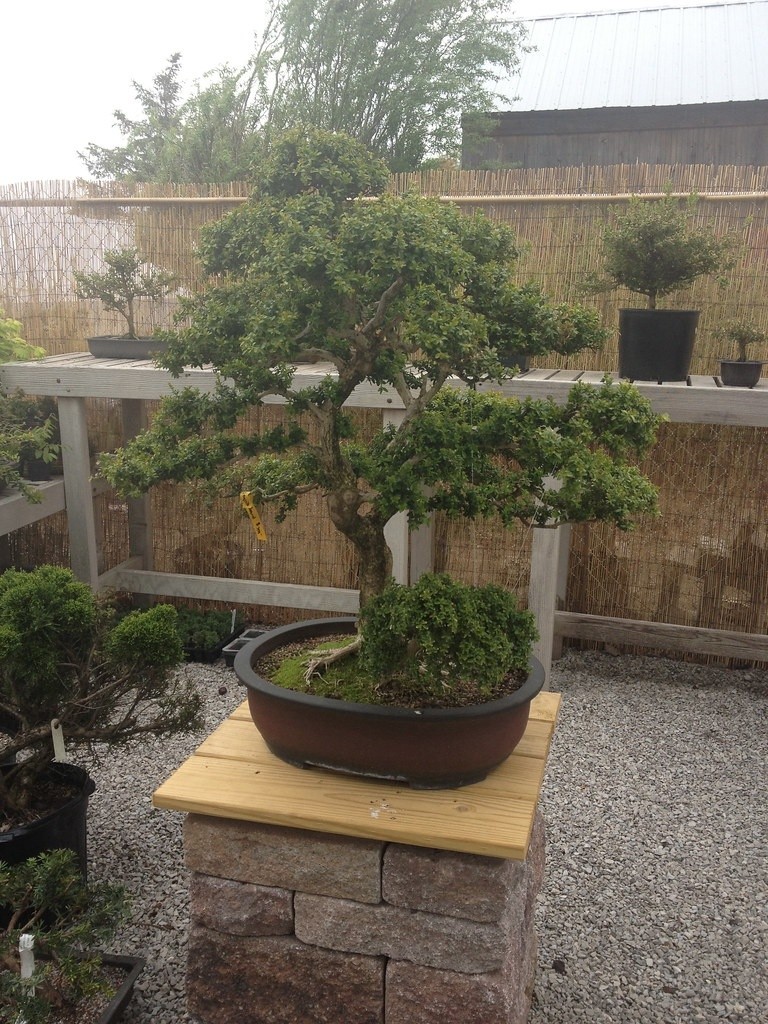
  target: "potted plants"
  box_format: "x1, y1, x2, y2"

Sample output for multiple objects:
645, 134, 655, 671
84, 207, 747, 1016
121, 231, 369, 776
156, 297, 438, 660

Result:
592, 178, 743, 382
177, 607, 247, 664
710, 317, 766, 387
72, 244, 189, 360
89, 121, 675, 793
0, 565, 199, 1024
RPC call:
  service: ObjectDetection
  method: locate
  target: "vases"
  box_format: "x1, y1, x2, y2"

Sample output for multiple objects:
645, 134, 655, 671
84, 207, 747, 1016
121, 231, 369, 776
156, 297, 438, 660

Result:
223, 627, 270, 667
